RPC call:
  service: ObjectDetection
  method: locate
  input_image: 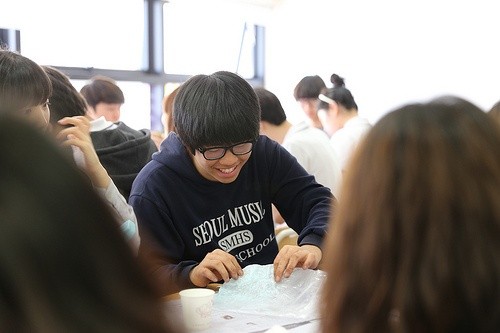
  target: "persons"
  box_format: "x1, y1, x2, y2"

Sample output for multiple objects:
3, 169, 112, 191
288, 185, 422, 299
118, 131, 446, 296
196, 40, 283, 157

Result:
0, 109, 179, 333
161, 87, 179, 135
129, 71, 337, 292
319, 95, 500, 333
253, 74, 371, 224
0, 51, 160, 266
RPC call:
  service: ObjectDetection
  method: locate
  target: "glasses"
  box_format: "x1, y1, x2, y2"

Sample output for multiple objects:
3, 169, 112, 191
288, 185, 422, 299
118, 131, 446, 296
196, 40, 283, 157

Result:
189, 139, 258, 160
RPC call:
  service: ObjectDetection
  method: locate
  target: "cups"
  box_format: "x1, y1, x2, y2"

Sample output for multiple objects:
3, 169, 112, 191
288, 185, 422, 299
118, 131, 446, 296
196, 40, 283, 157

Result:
179, 288, 215, 331
